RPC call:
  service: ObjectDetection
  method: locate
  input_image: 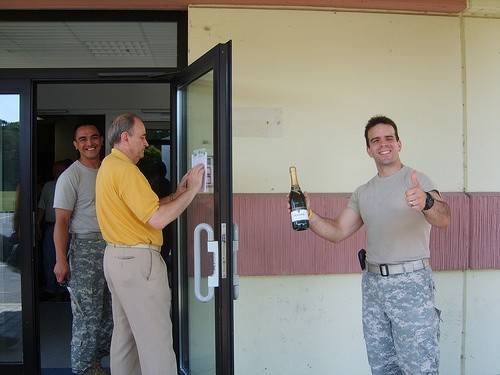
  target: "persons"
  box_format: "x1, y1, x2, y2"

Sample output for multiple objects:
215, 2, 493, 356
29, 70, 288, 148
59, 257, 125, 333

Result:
36, 159, 74, 302
286, 117, 450, 375
95, 114, 205, 375
53, 124, 114, 375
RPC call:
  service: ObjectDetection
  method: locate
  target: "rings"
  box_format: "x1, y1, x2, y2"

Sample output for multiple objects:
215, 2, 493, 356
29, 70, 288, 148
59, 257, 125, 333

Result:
410, 200, 413, 206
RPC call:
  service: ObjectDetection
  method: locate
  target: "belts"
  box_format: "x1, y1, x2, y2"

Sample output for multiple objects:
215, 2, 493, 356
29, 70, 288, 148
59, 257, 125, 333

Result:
75, 232, 103, 239
108, 243, 161, 252
368, 259, 429, 276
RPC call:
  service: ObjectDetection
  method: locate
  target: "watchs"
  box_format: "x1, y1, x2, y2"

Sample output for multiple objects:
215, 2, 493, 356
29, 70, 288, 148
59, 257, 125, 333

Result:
424, 192, 434, 210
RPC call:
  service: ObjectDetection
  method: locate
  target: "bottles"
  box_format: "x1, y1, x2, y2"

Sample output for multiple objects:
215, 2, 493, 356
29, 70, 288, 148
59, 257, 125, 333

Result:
289, 166, 310, 231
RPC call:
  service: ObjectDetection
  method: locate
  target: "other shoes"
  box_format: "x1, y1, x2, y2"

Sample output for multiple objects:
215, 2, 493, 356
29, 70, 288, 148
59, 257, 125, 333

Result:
39, 291, 56, 300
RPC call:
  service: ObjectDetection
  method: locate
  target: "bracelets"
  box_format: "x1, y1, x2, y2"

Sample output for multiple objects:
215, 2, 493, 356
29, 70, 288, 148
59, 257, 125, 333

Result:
169, 193, 173, 201
308, 209, 312, 218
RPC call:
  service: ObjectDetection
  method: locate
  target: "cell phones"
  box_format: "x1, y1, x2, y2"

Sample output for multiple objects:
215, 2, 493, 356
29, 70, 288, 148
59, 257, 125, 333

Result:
359, 248, 366, 270
